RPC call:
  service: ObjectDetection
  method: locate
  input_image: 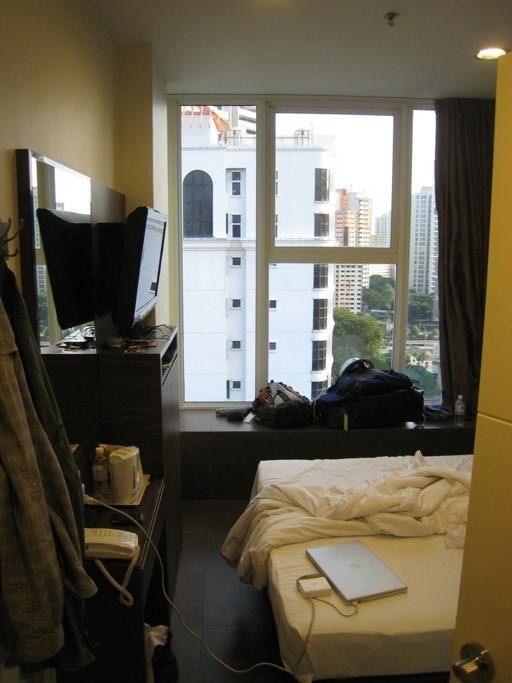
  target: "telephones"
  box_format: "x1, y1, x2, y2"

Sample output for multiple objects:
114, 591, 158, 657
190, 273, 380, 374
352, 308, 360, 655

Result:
84, 528, 138, 559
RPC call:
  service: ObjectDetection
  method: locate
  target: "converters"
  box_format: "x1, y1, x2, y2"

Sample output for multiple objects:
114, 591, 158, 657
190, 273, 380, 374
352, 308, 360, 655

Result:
297, 576, 331, 598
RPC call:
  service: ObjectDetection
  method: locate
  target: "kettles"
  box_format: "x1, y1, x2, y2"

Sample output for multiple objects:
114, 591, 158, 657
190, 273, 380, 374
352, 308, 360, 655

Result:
107, 445, 142, 505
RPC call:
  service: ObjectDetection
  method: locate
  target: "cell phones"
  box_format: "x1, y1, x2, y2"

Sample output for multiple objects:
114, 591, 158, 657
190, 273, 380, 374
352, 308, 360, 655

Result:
111, 512, 144, 526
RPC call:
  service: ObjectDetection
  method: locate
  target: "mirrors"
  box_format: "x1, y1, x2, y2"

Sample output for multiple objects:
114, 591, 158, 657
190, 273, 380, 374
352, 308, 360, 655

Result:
15, 148, 126, 353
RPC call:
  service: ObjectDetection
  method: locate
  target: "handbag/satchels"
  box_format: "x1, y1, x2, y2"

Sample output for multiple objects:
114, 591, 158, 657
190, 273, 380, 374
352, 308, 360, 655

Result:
253, 380, 313, 427
314, 369, 424, 428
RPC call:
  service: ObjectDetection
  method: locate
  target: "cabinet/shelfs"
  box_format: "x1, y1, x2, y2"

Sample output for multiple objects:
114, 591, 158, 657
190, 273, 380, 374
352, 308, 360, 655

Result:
57, 477, 170, 683
39, 325, 182, 567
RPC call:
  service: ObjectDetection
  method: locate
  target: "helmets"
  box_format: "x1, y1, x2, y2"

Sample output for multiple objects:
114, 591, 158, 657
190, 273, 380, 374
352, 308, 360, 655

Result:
339, 357, 374, 375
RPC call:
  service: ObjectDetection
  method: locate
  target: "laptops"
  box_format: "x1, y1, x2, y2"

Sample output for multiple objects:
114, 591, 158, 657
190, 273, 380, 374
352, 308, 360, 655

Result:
305, 539, 408, 605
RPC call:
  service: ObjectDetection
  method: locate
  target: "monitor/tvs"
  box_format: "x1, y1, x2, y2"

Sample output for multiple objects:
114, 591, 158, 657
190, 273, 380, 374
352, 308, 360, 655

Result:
36, 207, 94, 331
95, 206, 168, 330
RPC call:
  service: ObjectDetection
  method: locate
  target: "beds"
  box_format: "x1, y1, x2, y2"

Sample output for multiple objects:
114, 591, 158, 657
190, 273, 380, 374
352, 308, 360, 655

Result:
219, 454, 473, 683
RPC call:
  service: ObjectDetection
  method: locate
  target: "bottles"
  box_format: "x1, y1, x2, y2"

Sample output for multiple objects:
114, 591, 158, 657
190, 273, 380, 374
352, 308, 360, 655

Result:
93, 447, 109, 499
455, 395, 465, 428
